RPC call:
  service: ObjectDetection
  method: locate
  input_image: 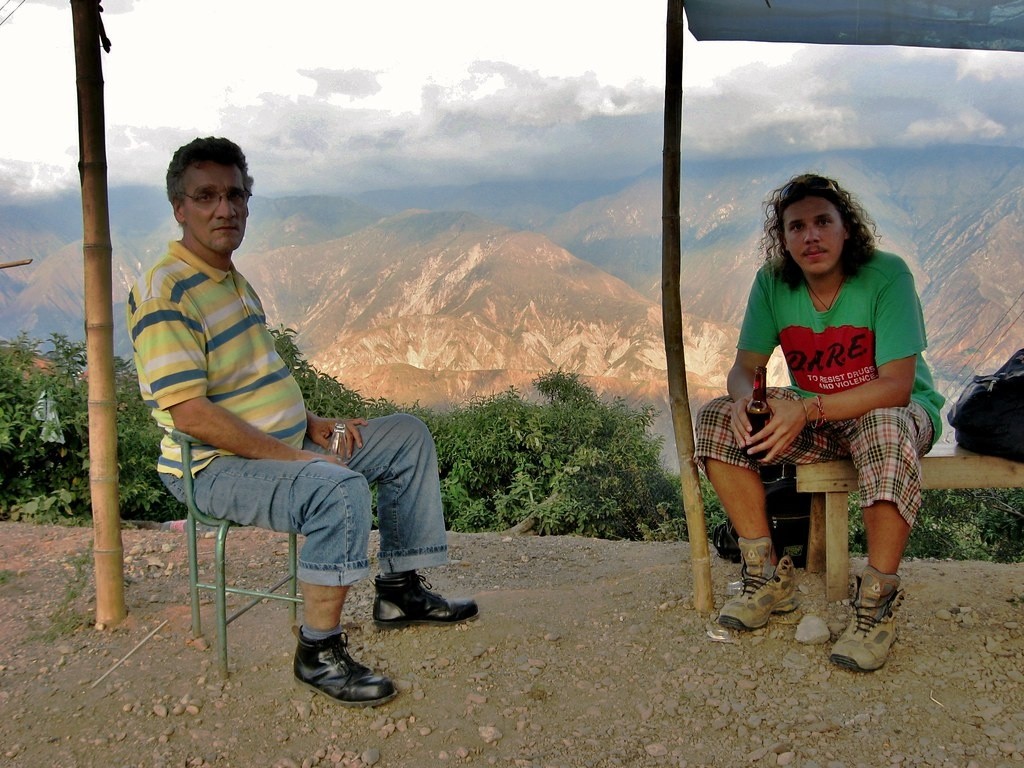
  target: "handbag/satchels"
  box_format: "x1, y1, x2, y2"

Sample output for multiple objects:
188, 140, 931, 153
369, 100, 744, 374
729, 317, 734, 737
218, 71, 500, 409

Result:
947, 348, 1024, 463
712, 465, 811, 570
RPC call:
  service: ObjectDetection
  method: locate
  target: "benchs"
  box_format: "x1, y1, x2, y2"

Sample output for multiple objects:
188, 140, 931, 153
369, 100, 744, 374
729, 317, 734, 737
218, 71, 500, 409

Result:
797, 443, 1024, 600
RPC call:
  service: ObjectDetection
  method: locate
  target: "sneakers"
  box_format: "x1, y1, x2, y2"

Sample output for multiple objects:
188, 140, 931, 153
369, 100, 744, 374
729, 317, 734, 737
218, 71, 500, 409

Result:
829, 565, 905, 672
719, 536, 798, 631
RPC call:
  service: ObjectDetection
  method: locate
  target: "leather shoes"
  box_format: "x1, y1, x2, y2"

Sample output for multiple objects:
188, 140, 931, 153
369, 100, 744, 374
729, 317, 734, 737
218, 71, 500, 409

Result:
293, 625, 397, 708
372, 569, 479, 629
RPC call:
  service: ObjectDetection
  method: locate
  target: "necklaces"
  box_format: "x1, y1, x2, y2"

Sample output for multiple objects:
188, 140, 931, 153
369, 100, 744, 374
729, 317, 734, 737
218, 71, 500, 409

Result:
805, 273, 844, 310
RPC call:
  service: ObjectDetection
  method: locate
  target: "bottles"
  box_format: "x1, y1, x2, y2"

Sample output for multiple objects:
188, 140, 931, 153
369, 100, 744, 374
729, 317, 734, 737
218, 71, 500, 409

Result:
327, 422, 348, 464
741, 366, 770, 459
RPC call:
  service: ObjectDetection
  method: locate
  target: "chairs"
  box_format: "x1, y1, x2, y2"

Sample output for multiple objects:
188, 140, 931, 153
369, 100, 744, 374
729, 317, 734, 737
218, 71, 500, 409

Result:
171, 428, 305, 680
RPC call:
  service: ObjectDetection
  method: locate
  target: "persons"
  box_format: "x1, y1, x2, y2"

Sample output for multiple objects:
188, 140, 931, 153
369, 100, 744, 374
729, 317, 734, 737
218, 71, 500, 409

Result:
126, 136, 480, 704
689, 172, 945, 672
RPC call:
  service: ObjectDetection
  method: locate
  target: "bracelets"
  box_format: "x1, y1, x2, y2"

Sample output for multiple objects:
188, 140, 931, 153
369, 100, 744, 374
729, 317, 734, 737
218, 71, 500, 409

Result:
798, 393, 826, 428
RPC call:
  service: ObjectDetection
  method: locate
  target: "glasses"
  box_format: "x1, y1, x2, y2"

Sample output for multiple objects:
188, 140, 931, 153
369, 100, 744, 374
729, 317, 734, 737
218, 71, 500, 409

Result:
183, 185, 252, 209
779, 175, 859, 226
